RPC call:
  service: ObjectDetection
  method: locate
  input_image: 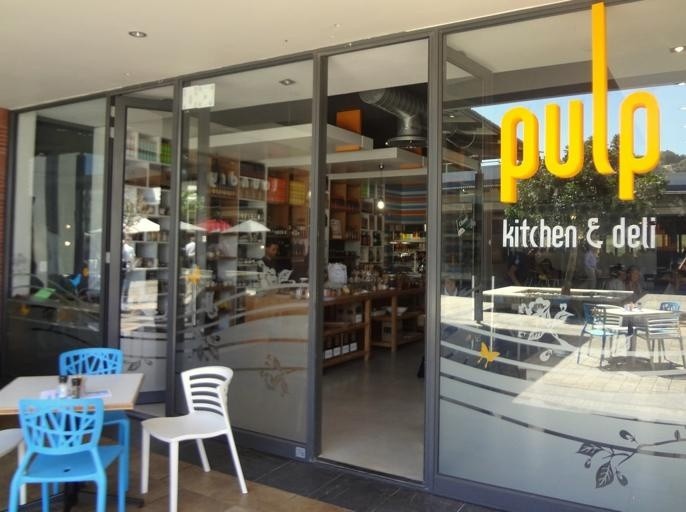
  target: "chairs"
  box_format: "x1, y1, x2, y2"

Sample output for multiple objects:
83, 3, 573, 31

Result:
7, 347, 248, 512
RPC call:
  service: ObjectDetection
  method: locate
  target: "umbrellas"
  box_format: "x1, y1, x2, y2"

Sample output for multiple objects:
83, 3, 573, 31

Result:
84, 212, 270, 236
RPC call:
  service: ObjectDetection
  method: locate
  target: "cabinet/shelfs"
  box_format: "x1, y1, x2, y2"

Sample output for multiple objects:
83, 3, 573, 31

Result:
120, 126, 385, 351
244, 282, 426, 371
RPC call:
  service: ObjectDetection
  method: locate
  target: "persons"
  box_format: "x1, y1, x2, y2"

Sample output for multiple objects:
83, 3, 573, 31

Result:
121, 237, 136, 305
185, 236, 195, 267
250, 241, 284, 283
441, 273, 459, 297
623, 266, 642, 294
576, 239, 601, 294
537, 256, 562, 289
604, 263, 625, 291
507, 234, 543, 287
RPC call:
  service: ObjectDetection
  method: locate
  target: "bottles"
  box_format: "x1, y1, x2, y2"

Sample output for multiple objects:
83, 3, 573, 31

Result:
72, 377, 81, 399
58, 375, 68, 398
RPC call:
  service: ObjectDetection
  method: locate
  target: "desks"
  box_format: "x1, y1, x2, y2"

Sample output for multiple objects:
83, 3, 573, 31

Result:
0, 373, 145, 508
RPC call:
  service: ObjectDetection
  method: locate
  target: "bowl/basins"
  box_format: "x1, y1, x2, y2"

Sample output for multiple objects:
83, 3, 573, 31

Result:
387, 307, 408, 317
